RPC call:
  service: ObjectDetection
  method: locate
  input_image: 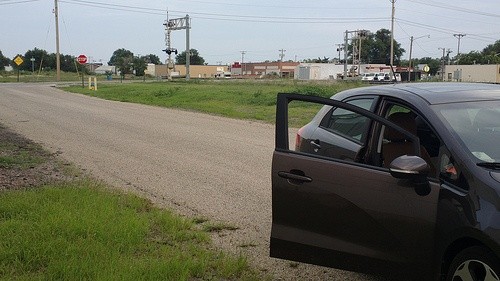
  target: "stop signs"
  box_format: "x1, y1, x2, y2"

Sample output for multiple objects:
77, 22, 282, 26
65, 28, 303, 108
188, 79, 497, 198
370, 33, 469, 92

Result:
77, 54, 87, 64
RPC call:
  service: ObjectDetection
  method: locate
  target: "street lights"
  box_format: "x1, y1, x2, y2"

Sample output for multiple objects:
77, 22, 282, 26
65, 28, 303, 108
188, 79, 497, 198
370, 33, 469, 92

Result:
407, 34, 431, 82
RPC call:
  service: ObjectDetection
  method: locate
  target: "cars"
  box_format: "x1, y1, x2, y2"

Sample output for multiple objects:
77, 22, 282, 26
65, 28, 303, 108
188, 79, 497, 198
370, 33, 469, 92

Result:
267, 80, 500, 281
